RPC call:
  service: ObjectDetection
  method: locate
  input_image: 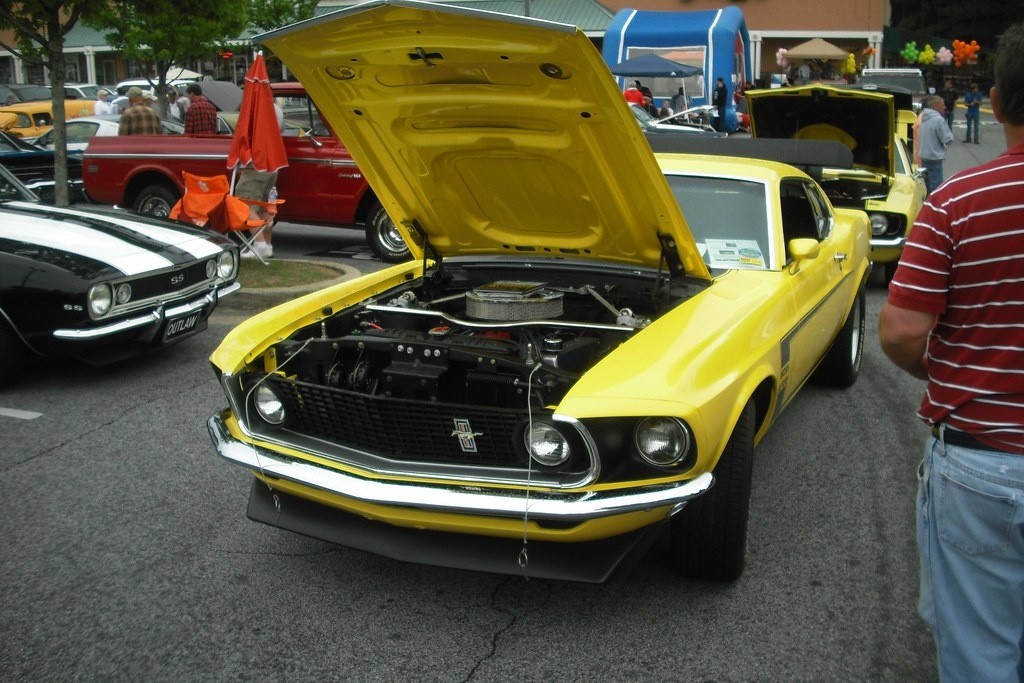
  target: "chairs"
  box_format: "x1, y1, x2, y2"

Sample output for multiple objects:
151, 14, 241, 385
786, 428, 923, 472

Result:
166, 170, 287, 266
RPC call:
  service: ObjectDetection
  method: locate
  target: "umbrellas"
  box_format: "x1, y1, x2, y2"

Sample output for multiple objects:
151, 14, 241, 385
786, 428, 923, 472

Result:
223, 50, 289, 196
153, 66, 204, 80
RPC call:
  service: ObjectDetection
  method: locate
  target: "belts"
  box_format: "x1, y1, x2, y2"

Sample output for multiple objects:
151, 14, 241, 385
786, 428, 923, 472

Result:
931, 426, 1007, 452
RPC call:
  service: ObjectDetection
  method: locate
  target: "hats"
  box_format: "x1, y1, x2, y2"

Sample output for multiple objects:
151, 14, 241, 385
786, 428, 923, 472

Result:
125, 87, 142, 97
97, 89, 109, 95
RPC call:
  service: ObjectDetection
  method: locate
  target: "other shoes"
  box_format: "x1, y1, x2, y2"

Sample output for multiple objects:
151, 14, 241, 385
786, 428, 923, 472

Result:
963, 138, 971, 143
266, 243, 272, 257
974, 139, 979, 144
240, 241, 265, 259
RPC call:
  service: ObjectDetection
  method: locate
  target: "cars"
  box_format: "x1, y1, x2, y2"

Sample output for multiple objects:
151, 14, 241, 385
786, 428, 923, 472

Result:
0, 164, 241, 392
206, 2, 874, 583
626, 68, 935, 287
0, 80, 312, 206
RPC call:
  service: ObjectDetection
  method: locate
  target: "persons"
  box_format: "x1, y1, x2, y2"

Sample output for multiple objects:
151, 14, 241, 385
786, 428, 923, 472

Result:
785, 58, 811, 86
164, 89, 186, 124
233, 85, 285, 259
184, 83, 217, 134
92, 89, 112, 115
177, 91, 191, 111
622, 79, 692, 122
962, 84, 983, 144
117, 86, 163, 136
935, 80, 958, 133
879, 24, 1024, 681
112, 86, 131, 110
711, 77, 729, 138
742, 80, 757, 96
910, 93, 954, 192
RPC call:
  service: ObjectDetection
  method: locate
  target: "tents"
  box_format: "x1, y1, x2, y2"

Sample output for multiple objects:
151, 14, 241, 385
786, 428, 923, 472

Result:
780, 37, 849, 86
609, 52, 709, 123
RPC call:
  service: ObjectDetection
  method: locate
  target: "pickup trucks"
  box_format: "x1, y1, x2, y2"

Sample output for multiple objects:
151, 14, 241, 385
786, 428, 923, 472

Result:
82, 84, 415, 265
